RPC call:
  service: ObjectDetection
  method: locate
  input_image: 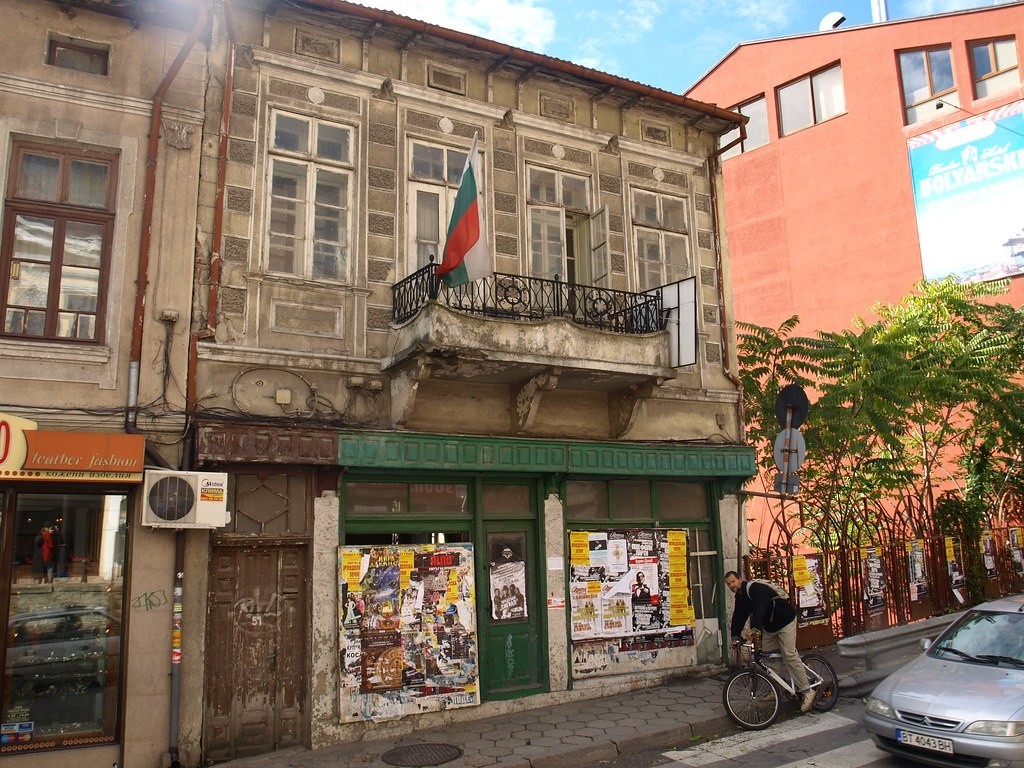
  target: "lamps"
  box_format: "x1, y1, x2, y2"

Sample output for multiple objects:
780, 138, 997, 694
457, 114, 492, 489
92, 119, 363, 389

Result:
235, 47, 256, 67
494, 110, 514, 129
597, 134, 621, 157
936, 100, 944, 109
372, 78, 400, 103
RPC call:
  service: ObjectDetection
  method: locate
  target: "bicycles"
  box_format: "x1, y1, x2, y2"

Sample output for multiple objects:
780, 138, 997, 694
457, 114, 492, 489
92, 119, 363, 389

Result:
721, 632, 839, 731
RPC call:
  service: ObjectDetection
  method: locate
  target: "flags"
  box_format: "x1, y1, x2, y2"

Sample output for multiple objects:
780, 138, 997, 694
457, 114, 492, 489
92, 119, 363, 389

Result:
438, 150, 494, 287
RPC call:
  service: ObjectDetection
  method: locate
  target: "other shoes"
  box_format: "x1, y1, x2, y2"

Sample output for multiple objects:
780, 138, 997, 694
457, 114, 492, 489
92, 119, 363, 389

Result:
760, 690, 775, 702
801, 689, 816, 712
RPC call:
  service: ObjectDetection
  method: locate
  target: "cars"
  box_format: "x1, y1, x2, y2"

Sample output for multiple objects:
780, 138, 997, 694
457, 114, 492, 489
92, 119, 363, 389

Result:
3, 602, 122, 682
863, 592, 1024, 768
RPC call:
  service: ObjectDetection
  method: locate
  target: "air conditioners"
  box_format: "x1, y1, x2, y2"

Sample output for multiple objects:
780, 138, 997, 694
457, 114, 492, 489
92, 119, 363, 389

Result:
139, 469, 228, 530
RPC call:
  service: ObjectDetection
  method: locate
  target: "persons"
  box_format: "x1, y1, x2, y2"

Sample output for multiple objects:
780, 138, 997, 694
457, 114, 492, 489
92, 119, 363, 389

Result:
494, 585, 524, 618
34, 520, 67, 584
632, 572, 650, 603
345, 599, 357, 624
724, 571, 815, 713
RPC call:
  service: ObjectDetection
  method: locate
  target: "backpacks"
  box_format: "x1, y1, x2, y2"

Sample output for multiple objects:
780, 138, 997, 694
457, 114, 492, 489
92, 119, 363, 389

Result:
746, 579, 790, 621
